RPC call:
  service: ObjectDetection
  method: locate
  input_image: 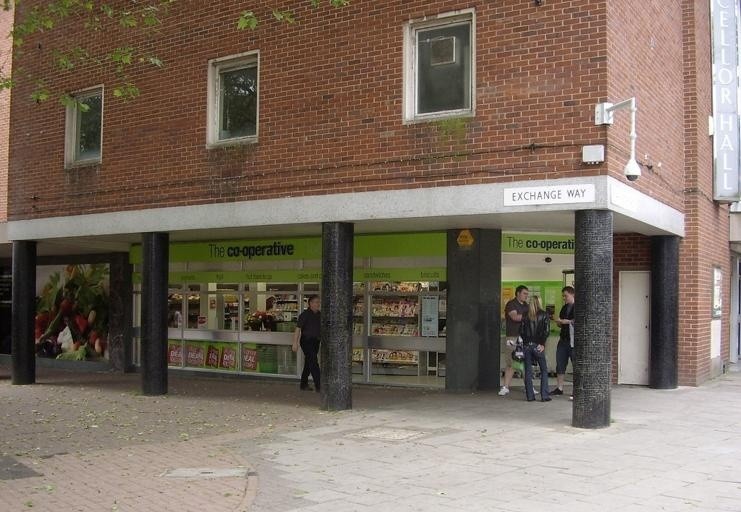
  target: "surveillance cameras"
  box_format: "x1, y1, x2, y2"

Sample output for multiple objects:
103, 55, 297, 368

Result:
623, 158, 641, 181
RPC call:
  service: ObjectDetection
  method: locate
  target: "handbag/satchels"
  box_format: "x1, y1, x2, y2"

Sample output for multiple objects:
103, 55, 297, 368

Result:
512, 347, 525, 361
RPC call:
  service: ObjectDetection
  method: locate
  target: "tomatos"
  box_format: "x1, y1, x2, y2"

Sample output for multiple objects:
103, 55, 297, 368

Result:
74, 315, 87, 332
60, 299, 72, 317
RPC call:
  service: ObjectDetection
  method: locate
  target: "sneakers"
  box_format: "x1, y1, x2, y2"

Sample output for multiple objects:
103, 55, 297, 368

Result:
533, 387, 563, 402
301, 386, 313, 391
498, 387, 509, 395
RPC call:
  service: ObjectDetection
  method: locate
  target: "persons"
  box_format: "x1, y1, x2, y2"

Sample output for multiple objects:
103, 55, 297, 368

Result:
292, 294, 321, 390
263, 302, 277, 332
519, 295, 553, 401
498, 285, 539, 396
550, 286, 575, 400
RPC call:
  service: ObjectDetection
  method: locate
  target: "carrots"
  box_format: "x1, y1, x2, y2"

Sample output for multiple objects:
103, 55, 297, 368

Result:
34, 306, 56, 338
